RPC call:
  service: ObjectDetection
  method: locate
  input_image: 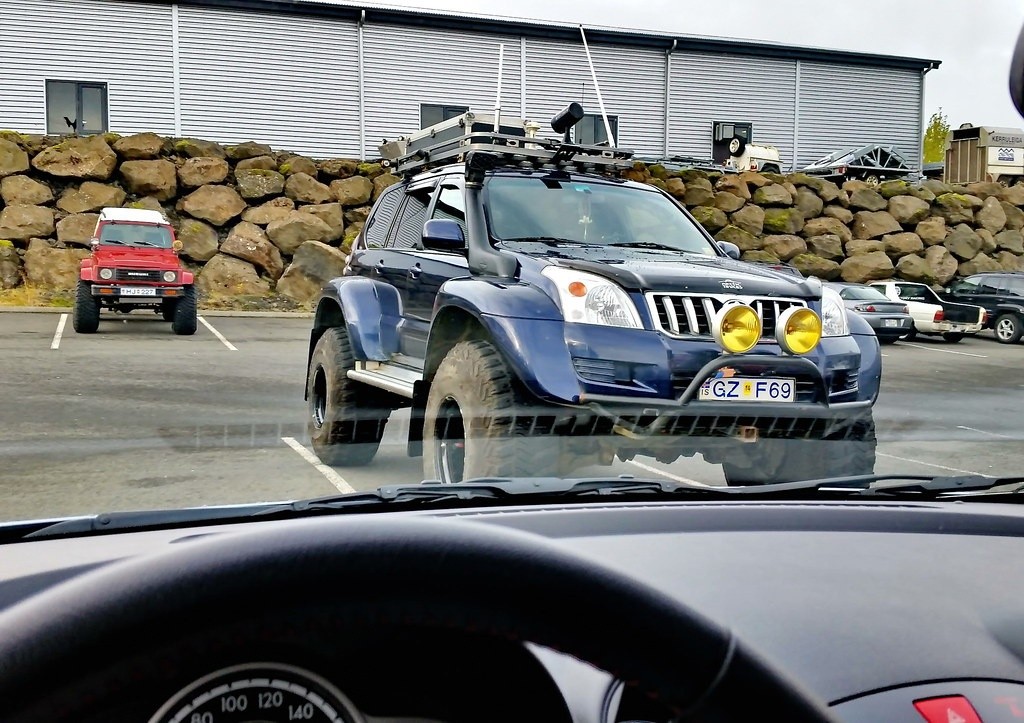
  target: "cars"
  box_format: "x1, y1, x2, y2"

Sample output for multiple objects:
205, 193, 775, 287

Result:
922, 163, 944, 180
807, 150, 910, 190
823, 282, 914, 345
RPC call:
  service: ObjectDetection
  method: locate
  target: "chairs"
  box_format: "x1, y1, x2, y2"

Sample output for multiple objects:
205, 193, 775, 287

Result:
145, 231, 164, 243
104, 228, 124, 244
575, 201, 633, 242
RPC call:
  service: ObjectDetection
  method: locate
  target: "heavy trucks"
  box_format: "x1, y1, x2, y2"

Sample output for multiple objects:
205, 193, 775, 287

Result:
943, 121, 1024, 188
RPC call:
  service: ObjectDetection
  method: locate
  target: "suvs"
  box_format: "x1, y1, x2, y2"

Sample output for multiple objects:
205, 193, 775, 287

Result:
73, 208, 198, 335
936, 270, 1024, 344
303, 132, 885, 490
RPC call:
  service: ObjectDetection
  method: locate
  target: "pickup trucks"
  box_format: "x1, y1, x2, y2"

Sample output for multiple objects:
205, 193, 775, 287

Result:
866, 280, 988, 343
